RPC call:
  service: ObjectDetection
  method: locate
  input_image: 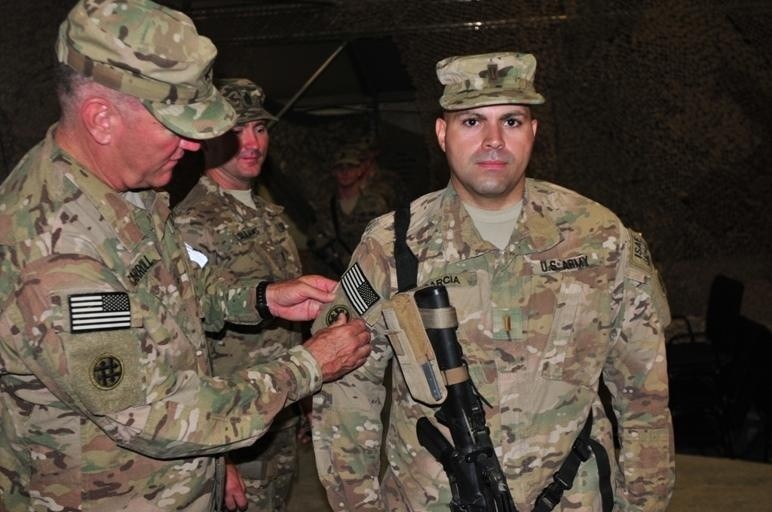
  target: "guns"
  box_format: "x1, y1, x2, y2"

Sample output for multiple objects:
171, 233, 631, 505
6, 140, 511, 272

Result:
414, 287, 516, 510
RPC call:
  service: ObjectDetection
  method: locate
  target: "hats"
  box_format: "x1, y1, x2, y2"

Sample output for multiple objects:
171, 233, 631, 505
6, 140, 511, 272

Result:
51, 0, 238, 143
210, 77, 278, 124
330, 147, 359, 168
434, 50, 548, 111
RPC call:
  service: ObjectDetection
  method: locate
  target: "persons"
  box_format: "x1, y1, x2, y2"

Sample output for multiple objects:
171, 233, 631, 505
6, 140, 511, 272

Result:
170, 77, 310, 510
307, 149, 389, 277
0, 0, 374, 511
309, 51, 678, 512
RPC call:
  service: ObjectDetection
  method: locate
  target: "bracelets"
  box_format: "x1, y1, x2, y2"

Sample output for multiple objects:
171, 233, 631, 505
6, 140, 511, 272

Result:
255, 280, 274, 322
